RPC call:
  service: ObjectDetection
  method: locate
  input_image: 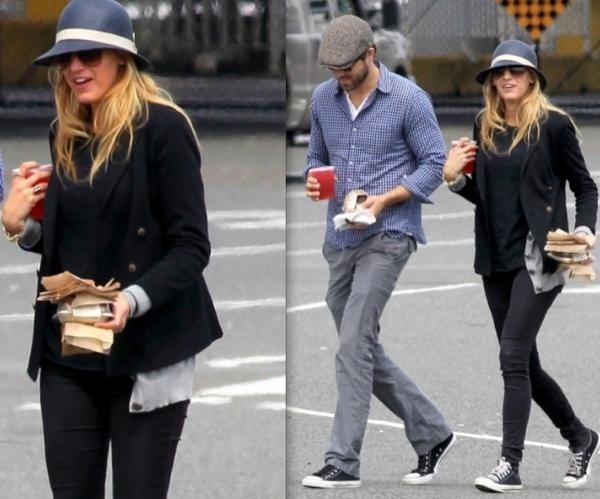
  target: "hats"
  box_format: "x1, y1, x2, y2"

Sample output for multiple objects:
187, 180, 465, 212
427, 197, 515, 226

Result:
475, 40, 547, 92
319, 14, 374, 70
32, 0, 150, 70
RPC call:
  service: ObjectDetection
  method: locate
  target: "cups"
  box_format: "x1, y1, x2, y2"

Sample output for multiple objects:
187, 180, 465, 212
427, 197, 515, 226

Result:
451, 141, 477, 176
309, 167, 337, 200
12, 165, 54, 222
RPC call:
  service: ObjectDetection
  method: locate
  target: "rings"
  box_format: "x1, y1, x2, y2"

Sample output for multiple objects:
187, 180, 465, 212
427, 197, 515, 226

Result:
33, 185, 40, 193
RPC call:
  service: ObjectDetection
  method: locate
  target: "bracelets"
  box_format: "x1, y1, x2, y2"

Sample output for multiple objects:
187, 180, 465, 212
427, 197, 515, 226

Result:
443, 179, 456, 186
2, 224, 23, 241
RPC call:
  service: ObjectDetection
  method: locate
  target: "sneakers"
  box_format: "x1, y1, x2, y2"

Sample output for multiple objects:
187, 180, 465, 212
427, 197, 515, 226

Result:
561, 429, 600, 488
474, 457, 523, 493
404, 432, 456, 485
302, 465, 361, 490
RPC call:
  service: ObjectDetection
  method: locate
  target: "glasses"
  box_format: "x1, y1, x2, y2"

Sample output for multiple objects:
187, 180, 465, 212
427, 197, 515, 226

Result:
488, 65, 527, 78
49, 49, 102, 68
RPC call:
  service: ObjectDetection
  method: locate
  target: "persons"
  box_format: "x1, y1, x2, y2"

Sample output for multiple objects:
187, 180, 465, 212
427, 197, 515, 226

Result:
0, 0, 223, 499
443, 39, 599, 492
301, 14, 456, 488
